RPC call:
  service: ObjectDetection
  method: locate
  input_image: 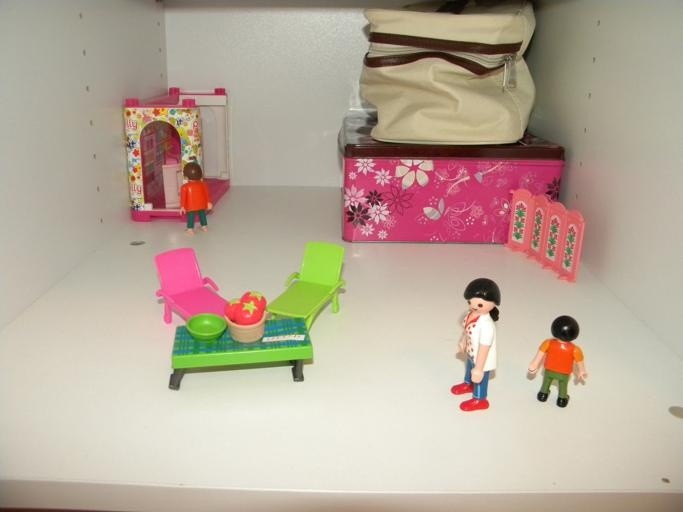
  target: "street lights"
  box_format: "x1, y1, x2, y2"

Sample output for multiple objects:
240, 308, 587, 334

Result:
359, 0, 537, 146
